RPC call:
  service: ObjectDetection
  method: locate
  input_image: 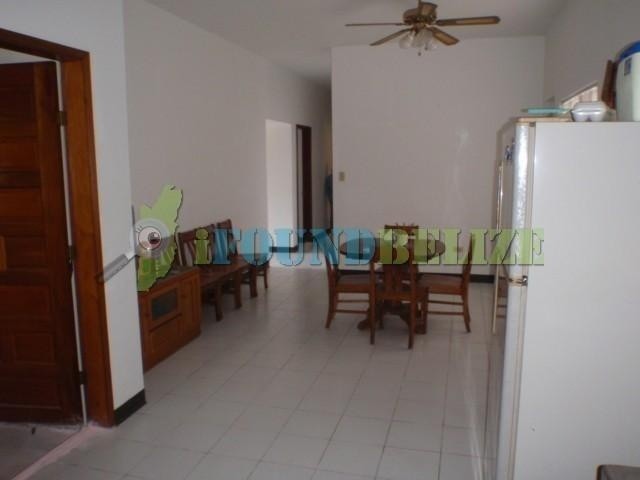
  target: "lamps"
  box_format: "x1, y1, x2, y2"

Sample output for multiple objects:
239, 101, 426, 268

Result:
400, 26, 439, 50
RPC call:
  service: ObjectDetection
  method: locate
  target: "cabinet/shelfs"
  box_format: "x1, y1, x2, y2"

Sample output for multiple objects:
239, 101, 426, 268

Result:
140, 265, 202, 373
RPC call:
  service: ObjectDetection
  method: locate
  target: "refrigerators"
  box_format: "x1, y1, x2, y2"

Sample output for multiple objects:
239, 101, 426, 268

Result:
482, 118, 636, 480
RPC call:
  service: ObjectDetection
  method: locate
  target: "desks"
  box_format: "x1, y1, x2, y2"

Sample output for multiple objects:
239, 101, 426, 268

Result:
337, 234, 446, 327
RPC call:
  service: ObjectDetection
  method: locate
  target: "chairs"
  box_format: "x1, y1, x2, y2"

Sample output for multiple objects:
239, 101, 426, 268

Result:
385, 225, 420, 240
370, 228, 427, 349
218, 218, 269, 297
313, 227, 385, 329
182, 230, 229, 322
195, 223, 254, 308
416, 233, 475, 335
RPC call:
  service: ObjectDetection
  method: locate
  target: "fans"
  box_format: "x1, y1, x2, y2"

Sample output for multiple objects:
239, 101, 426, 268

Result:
344, 0, 500, 52
